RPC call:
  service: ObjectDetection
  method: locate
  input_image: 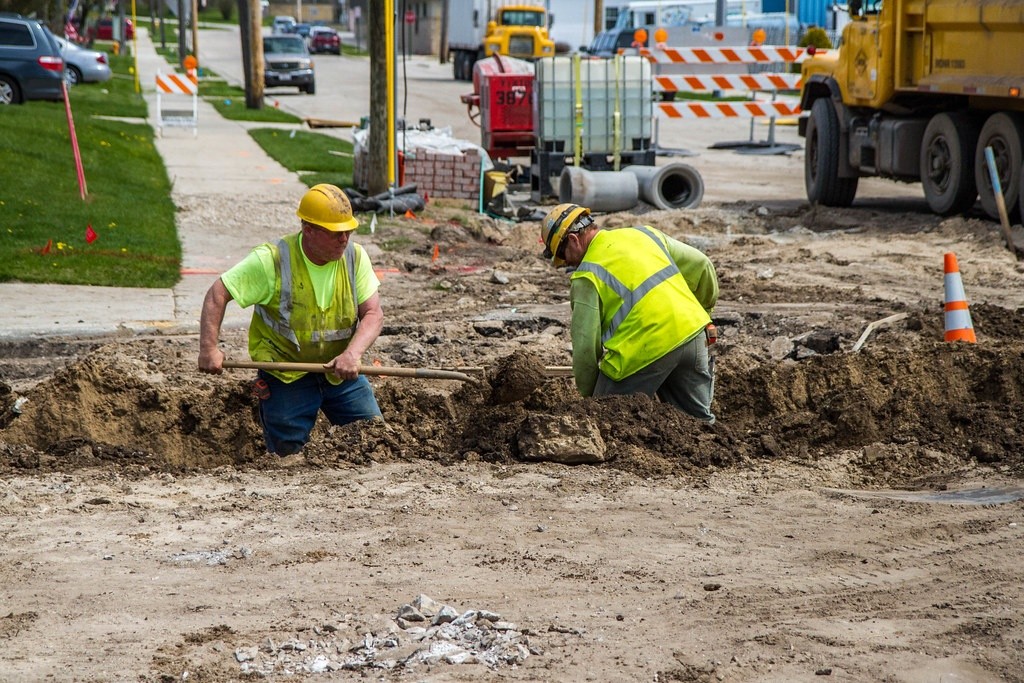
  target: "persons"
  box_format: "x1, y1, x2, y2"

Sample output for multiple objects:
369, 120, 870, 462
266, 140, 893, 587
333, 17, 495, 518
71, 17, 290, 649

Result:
197, 184, 385, 457
540, 201, 720, 429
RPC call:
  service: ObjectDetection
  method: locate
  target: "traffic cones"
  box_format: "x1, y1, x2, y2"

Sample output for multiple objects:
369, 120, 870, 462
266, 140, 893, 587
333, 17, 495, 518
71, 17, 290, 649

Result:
942, 253, 978, 345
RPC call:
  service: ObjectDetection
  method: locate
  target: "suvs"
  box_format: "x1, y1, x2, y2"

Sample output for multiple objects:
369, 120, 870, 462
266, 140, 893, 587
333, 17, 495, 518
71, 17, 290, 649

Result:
0, 10, 65, 106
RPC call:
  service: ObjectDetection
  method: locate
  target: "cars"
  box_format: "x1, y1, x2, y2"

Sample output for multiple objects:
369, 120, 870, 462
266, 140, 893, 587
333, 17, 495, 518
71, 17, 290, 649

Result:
52, 34, 113, 86
288, 23, 311, 38
72, 15, 135, 42
263, 33, 316, 95
307, 26, 342, 57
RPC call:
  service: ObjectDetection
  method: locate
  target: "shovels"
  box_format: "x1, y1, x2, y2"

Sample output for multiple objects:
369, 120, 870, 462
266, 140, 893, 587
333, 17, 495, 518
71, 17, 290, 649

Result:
221, 360, 547, 406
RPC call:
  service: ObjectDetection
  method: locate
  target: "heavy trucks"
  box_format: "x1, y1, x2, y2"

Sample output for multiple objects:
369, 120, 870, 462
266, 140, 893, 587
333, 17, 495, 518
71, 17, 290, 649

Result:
798, 0, 1023, 224
446, 0, 555, 81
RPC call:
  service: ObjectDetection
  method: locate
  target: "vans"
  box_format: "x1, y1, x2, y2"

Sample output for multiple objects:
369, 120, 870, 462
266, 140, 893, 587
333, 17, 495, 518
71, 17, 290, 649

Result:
273, 15, 297, 34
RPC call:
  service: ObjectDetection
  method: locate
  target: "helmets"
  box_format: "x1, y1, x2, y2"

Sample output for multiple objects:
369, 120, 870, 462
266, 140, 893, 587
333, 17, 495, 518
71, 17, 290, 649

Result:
541, 203, 590, 262
296, 183, 359, 232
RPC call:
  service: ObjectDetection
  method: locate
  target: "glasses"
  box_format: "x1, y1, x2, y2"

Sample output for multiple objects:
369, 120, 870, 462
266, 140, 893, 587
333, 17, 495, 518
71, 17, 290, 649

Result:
556, 237, 569, 260
311, 225, 353, 238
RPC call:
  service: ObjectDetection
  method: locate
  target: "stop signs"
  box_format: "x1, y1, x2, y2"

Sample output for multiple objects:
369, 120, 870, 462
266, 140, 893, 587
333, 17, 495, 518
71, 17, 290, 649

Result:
404, 9, 417, 24
